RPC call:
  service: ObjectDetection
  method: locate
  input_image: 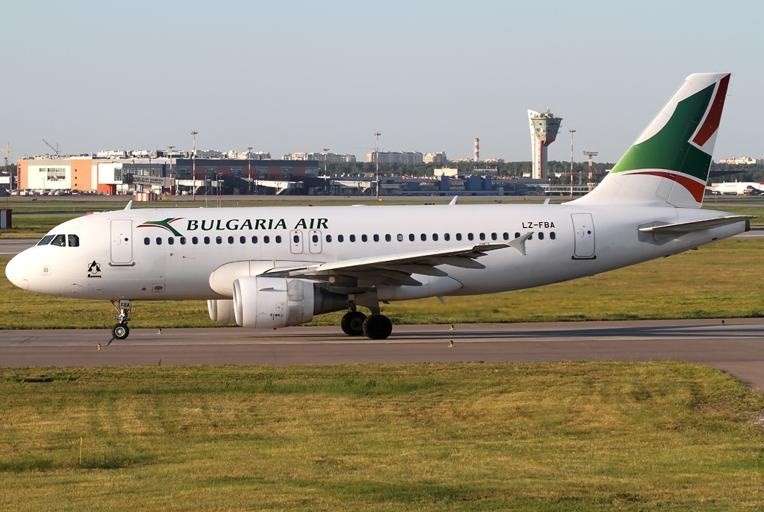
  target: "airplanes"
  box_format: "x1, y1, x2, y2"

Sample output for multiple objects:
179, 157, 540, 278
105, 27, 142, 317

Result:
2, 71, 757, 342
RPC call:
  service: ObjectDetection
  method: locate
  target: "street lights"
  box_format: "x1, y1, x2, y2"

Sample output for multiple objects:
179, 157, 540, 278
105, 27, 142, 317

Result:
246, 146, 254, 195
568, 127, 577, 200
321, 147, 329, 195
371, 131, 381, 200
188, 129, 197, 201
166, 144, 175, 201
581, 149, 598, 195
